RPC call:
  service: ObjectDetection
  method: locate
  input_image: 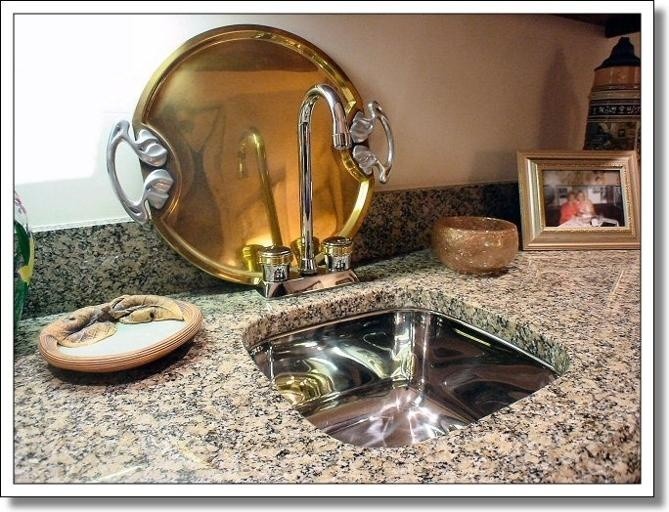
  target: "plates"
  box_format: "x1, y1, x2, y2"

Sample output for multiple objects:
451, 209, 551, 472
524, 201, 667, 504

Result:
38, 301, 203, 373
107, 27, 394, 285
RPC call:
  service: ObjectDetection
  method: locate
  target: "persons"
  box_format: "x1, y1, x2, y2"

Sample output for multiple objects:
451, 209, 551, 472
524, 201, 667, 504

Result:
559, 189, 596, 224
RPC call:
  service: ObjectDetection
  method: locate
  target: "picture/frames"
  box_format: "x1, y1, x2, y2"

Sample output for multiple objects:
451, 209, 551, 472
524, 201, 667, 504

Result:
516, 147, 642, 251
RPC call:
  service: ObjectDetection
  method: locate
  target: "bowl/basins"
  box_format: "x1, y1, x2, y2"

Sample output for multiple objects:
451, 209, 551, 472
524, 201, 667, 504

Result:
433, 218, 519, 275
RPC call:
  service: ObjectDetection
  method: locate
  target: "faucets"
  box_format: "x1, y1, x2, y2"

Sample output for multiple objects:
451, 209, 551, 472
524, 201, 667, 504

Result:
236, 127, 283, 246
297, 84, 351, 276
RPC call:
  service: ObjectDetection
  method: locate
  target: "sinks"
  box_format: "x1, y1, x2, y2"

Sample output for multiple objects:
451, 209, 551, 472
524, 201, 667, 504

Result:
248, 308, 560, 450
